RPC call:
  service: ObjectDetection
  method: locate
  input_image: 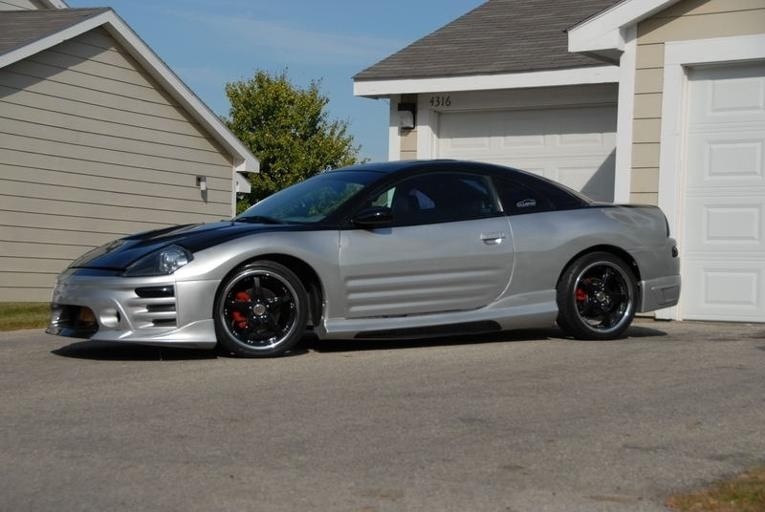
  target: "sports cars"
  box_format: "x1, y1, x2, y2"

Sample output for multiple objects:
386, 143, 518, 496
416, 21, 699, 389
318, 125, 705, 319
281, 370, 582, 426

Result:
44, 158, 682, 357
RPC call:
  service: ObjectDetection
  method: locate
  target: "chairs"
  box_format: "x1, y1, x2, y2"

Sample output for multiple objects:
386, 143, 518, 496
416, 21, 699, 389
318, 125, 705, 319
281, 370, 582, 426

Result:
424, 174, 480, 218
391, 185, 420, 216
